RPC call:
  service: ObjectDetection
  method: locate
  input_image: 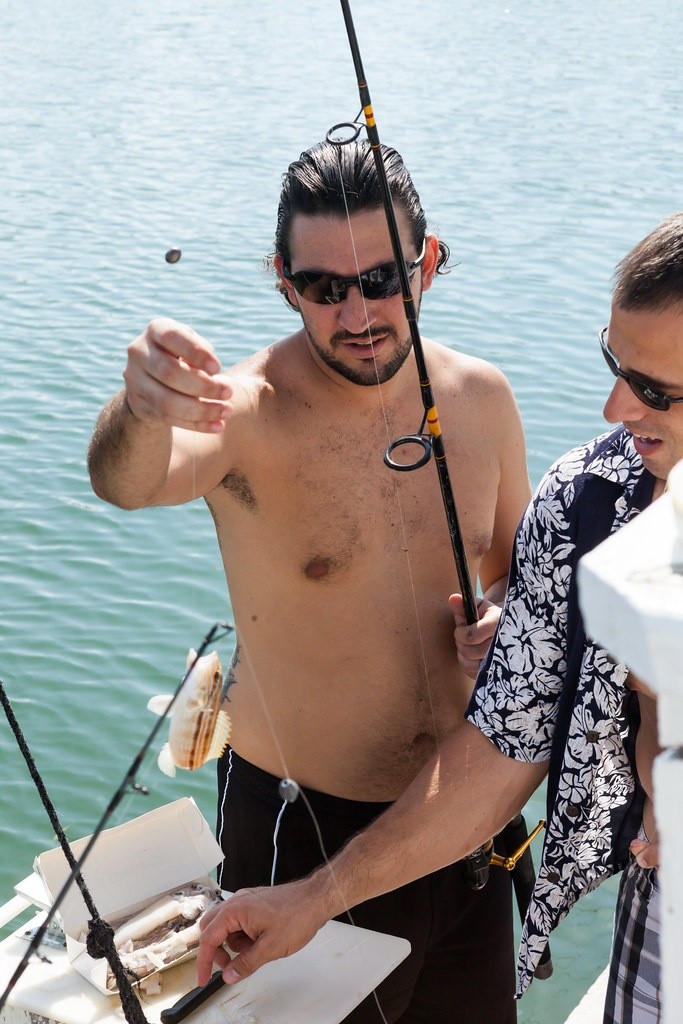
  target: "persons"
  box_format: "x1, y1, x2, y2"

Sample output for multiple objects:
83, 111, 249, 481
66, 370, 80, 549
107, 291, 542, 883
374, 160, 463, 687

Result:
88, 142, 533, 1024
197, 213, 683, 1024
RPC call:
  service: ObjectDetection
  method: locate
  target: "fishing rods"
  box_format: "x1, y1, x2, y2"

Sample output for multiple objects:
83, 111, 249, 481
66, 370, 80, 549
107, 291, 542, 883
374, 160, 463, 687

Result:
0, 619, 236, 1012
325, 1, 555, 980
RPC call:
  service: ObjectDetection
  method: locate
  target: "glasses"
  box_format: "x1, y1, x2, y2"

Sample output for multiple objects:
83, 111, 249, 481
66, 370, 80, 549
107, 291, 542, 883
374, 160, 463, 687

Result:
281, 236, 429, 305
598, 325, 683, 412
